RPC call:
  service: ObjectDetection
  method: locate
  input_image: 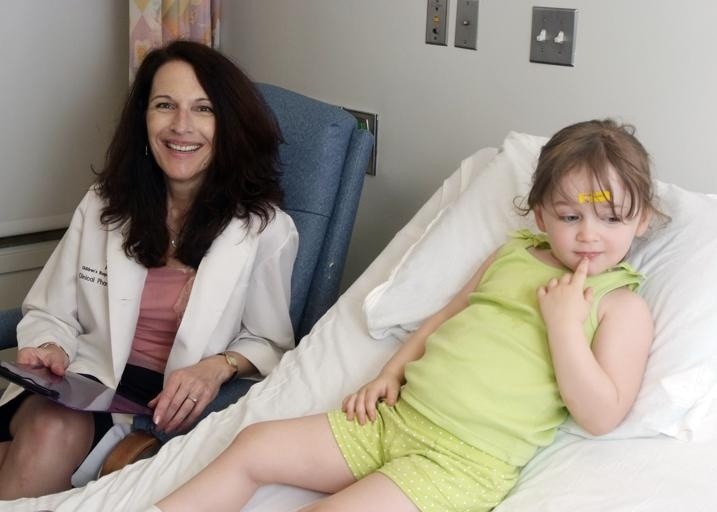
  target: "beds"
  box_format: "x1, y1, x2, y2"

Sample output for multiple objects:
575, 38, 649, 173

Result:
0, 131, 717, 512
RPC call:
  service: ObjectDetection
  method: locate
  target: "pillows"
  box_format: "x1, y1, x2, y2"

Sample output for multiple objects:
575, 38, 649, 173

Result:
365, 133, 717, 441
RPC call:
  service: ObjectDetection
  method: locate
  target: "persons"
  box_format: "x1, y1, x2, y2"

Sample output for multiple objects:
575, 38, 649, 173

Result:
0, 40, 299, 501
158, 118, 669, 512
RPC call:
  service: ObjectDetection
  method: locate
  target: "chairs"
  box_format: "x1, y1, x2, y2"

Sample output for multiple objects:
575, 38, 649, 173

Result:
0, 82, 375, 477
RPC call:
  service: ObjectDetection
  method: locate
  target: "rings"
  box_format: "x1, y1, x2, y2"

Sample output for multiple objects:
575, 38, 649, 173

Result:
188, 394, 197, 404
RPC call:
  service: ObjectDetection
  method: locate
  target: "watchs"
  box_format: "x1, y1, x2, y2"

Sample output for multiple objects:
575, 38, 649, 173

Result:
216, 351, 240, 385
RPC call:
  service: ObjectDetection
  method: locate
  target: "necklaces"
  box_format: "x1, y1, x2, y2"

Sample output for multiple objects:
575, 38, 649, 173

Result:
167, 224, 180, 247
